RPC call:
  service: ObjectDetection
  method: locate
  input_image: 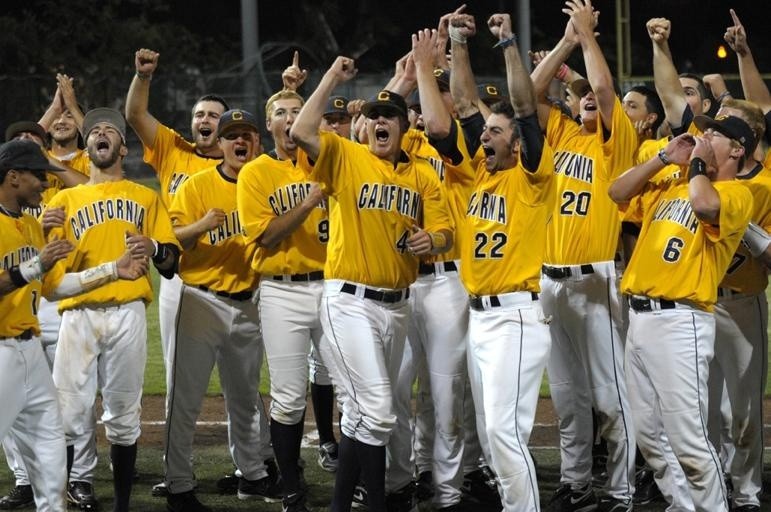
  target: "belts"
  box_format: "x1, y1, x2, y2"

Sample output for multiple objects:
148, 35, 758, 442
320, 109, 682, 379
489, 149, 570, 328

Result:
1, 328, 32, 340
419, 261, 457, 273
470, 291, 538, 309
199, 284, 252, 302
630, 297, 675, 311
341, 283, 410, 303
718, 287, 740, 297
543, 264, 595, 278
273, 271, 324, 281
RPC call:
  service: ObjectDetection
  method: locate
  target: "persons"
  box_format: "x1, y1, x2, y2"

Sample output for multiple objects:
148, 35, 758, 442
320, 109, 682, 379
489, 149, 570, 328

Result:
0, 0, 769, 511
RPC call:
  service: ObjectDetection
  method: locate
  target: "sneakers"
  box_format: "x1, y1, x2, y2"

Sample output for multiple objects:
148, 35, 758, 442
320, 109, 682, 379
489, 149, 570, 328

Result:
216, 459, 310, 512
318, 441, 339, 472
67, 481, 97, 511
108, 463, 140, 482
351, 466, 503, 512
1, 485, 35, 512
152, 472, 209, 512
544, 451, 663, 512
717, 454, 760, 512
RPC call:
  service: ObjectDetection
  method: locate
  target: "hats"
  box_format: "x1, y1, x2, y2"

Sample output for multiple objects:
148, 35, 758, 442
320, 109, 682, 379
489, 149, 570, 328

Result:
432, 69, 449, 87
573, 77, 622, 98
82, 108, 127, 144
477, 84, 503, 102
0, 141, 65, 172
322, 96, 349, 115
217, 109, 259, 137
361, 91, 408, 117
5, 121, 47, 144
693, 115, 753, 155
